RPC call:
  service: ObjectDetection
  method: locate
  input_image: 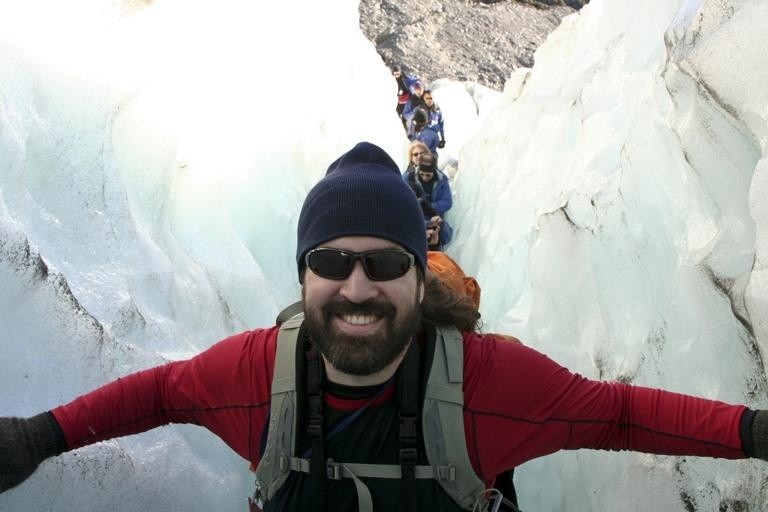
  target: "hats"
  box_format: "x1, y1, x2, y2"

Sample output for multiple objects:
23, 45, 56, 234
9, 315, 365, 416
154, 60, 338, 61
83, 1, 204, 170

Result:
413, 81, 423, 88
392, 65, 401, 72
412, 110, 426, 122
296, 142, 428, 271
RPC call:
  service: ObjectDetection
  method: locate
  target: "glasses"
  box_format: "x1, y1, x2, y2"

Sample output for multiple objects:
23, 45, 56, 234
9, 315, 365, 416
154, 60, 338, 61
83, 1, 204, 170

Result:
305, 247, 415, 281
411, 152, 424, 157
425, 98, 433, 100
420, 173, 430, 177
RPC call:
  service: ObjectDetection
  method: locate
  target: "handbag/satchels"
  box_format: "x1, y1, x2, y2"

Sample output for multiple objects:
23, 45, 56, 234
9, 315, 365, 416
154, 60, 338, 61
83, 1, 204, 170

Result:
438, 221, 454, 243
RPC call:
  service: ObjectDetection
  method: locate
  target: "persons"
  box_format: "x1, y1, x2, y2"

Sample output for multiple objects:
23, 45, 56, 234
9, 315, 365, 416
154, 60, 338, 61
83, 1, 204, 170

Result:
392, 70, 456, 250
0, 141, 768, 512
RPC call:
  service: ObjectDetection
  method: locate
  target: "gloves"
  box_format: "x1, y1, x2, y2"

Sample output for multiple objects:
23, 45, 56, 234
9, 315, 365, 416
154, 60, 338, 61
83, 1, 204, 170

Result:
438, 140, 446, 149
750, 409, 767, 461
0, 411, 63, 494
